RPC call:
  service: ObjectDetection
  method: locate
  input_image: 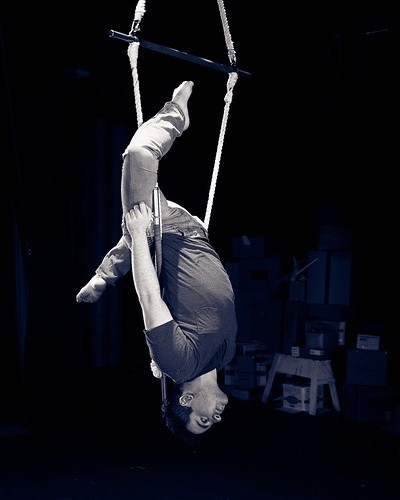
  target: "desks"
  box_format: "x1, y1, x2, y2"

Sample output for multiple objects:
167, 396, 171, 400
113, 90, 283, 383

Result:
261, 354, 342, 415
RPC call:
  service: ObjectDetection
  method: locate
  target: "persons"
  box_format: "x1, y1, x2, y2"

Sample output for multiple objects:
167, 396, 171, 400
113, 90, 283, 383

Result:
76, 80, 237, 441
